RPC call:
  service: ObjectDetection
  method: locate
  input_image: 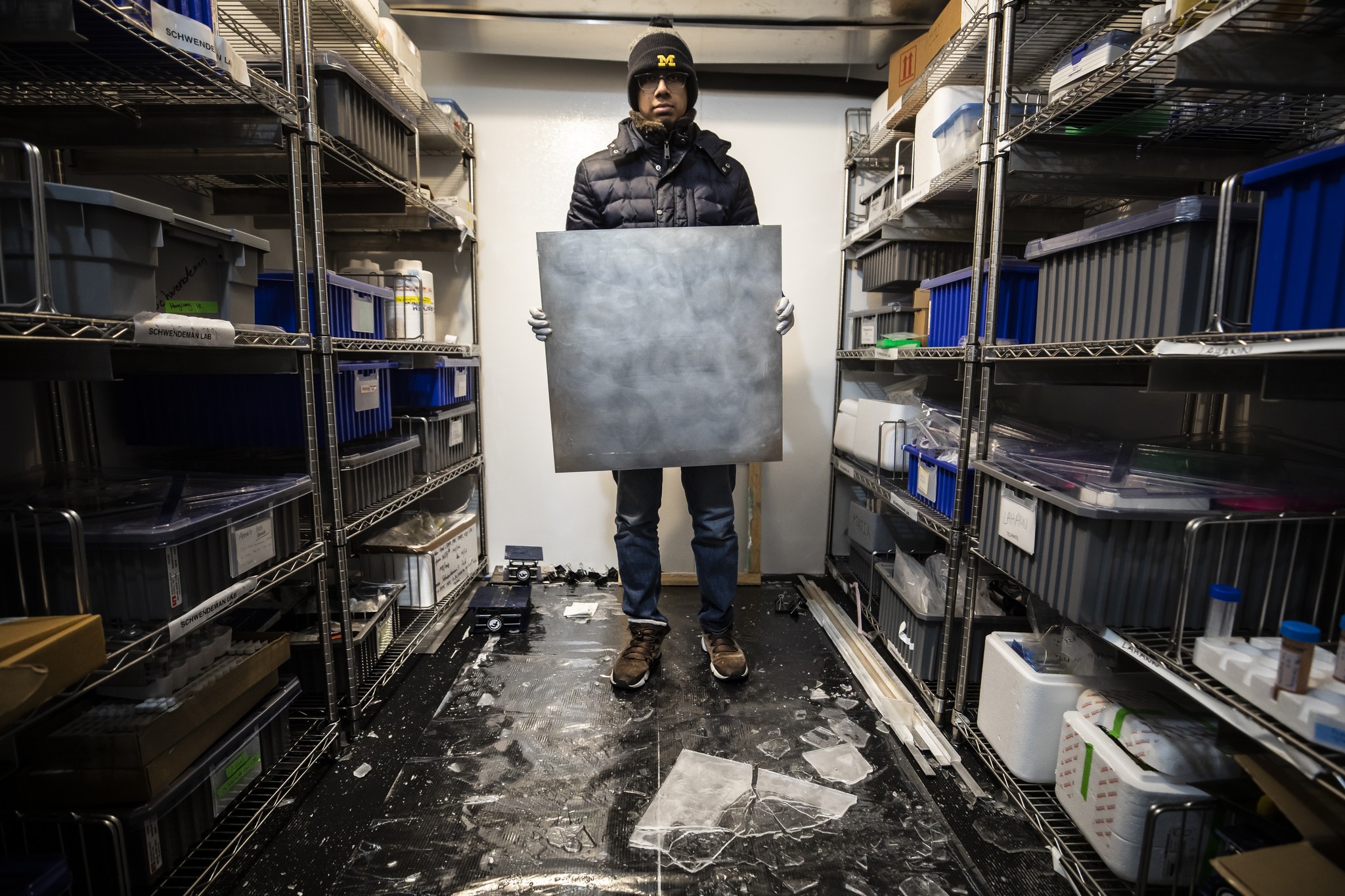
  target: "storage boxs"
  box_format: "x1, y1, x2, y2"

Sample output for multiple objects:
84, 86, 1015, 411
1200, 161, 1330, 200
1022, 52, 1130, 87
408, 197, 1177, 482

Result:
0, 0, 516, 896
834, 0, 1345, 896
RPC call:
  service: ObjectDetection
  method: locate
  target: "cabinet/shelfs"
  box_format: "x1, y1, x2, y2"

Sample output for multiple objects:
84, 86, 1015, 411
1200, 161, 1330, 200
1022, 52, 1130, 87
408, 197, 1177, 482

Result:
825, 0, 999, 726
957, 0, 1345, 896
0, 0, 342, 896
300, 0, 500, 747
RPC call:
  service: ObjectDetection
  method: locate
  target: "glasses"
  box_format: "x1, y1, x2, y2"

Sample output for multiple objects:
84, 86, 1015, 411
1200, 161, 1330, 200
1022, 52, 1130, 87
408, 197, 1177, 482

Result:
634, 71, 689, 90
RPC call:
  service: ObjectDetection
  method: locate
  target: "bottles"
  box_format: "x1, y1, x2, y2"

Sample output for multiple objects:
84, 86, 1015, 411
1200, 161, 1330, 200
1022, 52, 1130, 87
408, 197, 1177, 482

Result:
1272, 620, 1319, 699
1203, 584, 1240, 649
1333, 616, 1345, 684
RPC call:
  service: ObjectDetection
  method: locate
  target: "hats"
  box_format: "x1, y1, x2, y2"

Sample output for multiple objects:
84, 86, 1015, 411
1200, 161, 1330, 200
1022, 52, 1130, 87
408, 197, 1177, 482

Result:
627, 17, 698, 117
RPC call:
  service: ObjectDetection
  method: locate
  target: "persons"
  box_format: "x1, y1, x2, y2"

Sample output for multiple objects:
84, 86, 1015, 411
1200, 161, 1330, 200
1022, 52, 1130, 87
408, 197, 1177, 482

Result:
527, 34, 795, 689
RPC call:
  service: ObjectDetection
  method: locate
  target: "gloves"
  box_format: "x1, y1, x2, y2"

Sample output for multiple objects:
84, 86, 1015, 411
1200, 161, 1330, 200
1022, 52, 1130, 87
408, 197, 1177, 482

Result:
527, 307, 553, 342
776, 296, 794, 335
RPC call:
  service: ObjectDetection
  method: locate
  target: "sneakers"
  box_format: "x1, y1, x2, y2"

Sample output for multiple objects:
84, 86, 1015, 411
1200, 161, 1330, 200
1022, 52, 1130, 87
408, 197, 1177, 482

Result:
701, 622, 748, 679
611, 618, 672, 688
